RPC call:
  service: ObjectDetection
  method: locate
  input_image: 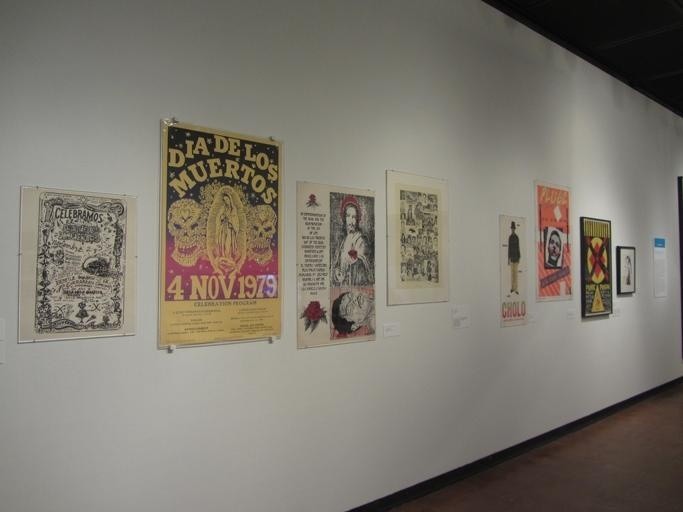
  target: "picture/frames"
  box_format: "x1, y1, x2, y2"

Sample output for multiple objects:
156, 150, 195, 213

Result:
616, 246, 635, 294
580, 216, 612, 318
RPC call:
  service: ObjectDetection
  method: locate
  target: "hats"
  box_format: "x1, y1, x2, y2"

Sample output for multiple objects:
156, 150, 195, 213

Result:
338, 194, 362, 226
508, 221, 518, 229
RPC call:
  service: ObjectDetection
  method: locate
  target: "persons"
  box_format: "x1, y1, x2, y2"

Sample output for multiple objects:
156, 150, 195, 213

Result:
502, 220, 522, 297
544, 229, 560, 267
215, 193, 240, 255
333, 199, 368, 286
330, 288, 375, 337
